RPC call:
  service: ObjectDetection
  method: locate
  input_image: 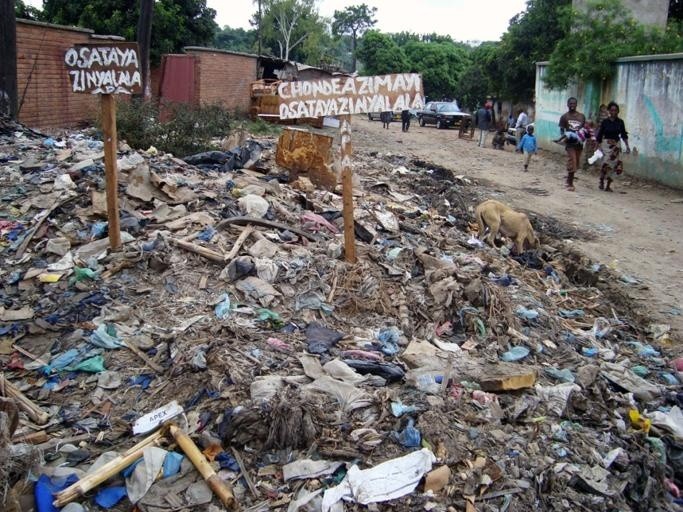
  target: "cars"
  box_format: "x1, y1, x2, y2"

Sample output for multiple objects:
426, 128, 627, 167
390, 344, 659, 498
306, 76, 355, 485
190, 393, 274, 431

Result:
416, 100, 471, 130
505, 122, 535, 145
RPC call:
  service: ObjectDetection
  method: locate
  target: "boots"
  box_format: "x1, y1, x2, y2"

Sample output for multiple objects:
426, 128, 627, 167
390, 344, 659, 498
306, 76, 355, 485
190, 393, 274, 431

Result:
567, 172, 576, 191
599, 176, 613, 192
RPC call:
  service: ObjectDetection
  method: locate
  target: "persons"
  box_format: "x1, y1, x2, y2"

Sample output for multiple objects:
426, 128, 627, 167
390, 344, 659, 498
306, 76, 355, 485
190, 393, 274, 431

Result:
558, 97, 590, 192
401, 110, 409, 132
475, 100, 536, 172
379, 111, 393, 129
551, 119, 596, 146
595, 101, 630, 192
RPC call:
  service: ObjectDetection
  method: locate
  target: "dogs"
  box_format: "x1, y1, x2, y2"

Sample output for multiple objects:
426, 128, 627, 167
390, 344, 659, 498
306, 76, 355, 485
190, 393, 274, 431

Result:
474, 199, 541, 255
